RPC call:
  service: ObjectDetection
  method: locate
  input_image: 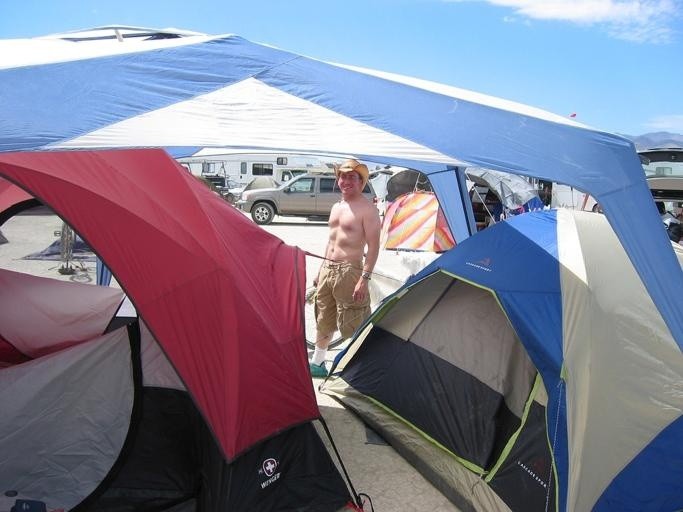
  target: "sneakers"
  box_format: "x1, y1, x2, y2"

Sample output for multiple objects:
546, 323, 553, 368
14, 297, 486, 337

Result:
309, 361, 328, 377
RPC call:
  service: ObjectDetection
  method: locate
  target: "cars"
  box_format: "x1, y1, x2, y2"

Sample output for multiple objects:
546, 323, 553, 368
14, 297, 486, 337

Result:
223, 188, 244, 204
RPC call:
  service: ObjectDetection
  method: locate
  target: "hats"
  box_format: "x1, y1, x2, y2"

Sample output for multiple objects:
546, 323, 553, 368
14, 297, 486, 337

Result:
333, 159, 369, 192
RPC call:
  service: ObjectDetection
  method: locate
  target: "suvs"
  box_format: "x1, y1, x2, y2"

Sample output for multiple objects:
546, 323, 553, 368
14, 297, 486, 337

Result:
240, 170, 378, 225
